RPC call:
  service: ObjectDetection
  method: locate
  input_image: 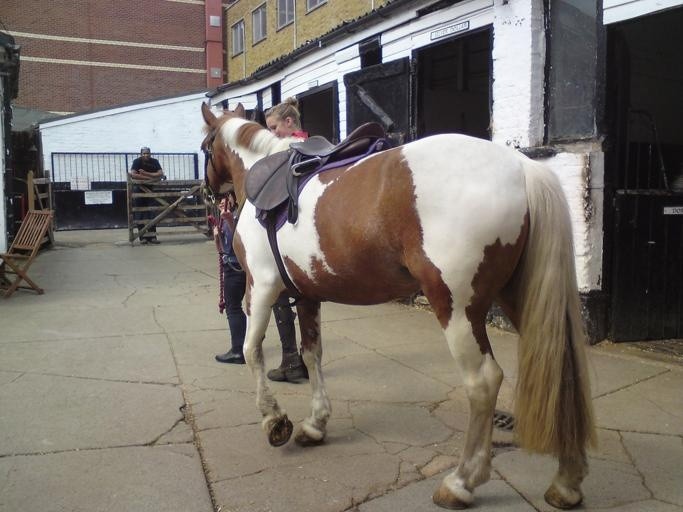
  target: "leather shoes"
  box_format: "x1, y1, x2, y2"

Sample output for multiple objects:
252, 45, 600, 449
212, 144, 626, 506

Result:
149, 237, 160, 244
268, 361, 304, 381
140, 238, 148, 244
216, 349, 245, 365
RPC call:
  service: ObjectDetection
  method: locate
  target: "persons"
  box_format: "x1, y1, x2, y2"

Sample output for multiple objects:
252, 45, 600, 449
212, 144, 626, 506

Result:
131, 146, 162, 244
212, 193, 247, 365
265, 96, 311, 383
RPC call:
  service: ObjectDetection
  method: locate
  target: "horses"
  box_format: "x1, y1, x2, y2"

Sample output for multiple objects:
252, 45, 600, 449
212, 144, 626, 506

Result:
200, 101, 599, 509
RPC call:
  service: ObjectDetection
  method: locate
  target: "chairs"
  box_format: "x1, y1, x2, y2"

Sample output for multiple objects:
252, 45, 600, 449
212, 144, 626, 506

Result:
0, 209, 54, 300
